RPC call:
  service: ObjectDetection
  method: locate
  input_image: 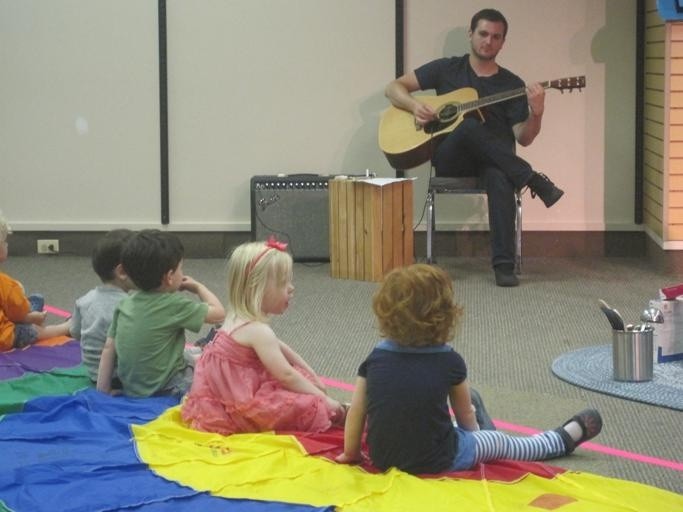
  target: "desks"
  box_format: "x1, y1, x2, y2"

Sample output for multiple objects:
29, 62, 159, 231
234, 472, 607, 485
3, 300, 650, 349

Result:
325, 177, 414, 284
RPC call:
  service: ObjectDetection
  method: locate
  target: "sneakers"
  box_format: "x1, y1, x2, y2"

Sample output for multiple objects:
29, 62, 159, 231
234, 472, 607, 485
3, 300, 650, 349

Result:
494, 261, 518, 285
527, 171, 563, 207
467, 389, 497, 430
558, 410, 601, 453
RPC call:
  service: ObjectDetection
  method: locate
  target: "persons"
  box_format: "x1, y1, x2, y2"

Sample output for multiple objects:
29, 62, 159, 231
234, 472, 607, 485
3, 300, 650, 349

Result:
178, 235, 354, 437
95, 228, 225, 401
334, 263, 603, 475
0, 214, 74, 354
376, 8, 565, 288
73, 227, 139, 391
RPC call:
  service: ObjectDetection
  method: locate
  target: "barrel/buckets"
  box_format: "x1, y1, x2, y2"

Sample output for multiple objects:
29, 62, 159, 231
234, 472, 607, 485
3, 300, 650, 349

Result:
613, 329, 654, 383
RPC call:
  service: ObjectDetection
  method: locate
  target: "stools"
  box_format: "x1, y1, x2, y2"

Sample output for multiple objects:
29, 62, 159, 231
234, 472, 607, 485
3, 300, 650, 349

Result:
425, 176, 524, 276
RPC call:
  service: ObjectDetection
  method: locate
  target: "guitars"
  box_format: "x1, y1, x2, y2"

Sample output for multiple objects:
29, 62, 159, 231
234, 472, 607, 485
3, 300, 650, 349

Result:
378, 76, 585, 169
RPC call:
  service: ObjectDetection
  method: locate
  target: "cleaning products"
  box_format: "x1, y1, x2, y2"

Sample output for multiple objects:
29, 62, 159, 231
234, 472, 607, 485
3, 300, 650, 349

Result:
646, 281, 683, 364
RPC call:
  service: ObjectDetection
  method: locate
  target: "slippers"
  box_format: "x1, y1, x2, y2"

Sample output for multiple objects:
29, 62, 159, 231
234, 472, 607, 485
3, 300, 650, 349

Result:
27, 294, 44, 312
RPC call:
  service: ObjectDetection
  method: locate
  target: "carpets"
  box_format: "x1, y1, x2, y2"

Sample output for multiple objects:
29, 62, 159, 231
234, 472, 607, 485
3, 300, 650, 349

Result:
552, 344, 683, 414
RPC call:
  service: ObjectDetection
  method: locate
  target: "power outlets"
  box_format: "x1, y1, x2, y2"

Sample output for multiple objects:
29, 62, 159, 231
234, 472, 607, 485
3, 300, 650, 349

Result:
38, 239, 59, 254
